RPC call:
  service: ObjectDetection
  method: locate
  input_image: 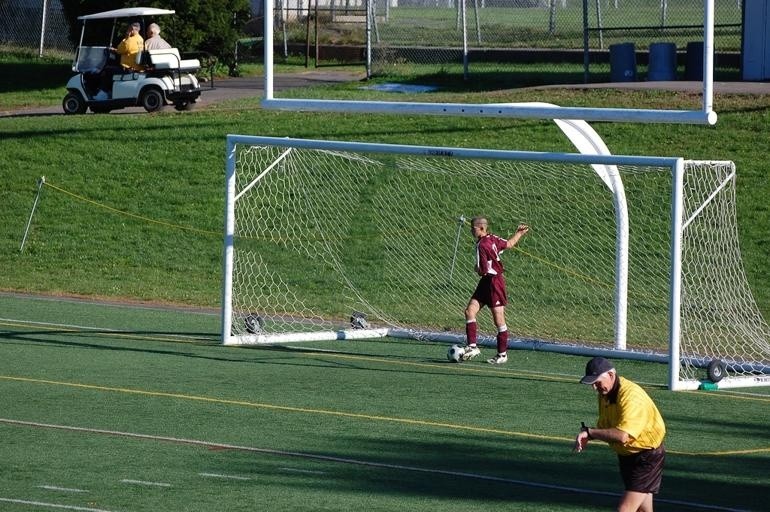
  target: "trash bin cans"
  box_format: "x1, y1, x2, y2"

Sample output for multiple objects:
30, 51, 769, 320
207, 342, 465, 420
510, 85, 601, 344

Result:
684, 42, 703, 81
608, 43, 636, 82
648, 42, 677, 81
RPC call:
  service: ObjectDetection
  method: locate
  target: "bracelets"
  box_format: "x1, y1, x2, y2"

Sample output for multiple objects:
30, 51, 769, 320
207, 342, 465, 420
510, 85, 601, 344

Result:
586, 427, 593, 441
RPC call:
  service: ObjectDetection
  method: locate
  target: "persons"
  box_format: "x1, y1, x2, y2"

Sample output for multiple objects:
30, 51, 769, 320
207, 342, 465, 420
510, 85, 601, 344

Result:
131, 22, 145, 54
573, 356, 666, 512
144, 23, 172, 65
91, 25, 142, 100
462, 217, 529, 364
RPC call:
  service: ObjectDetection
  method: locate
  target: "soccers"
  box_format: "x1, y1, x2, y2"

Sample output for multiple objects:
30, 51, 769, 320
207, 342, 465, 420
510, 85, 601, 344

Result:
447, 344, 465, 362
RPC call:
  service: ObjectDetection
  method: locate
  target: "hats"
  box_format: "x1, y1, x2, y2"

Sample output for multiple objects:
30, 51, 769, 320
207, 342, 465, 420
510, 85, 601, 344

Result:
579, 356, 616, 385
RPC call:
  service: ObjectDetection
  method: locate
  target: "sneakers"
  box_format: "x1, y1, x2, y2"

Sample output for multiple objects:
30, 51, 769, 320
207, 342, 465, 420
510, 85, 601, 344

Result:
459, 344, 481, 361
486, 352, 508, 364
